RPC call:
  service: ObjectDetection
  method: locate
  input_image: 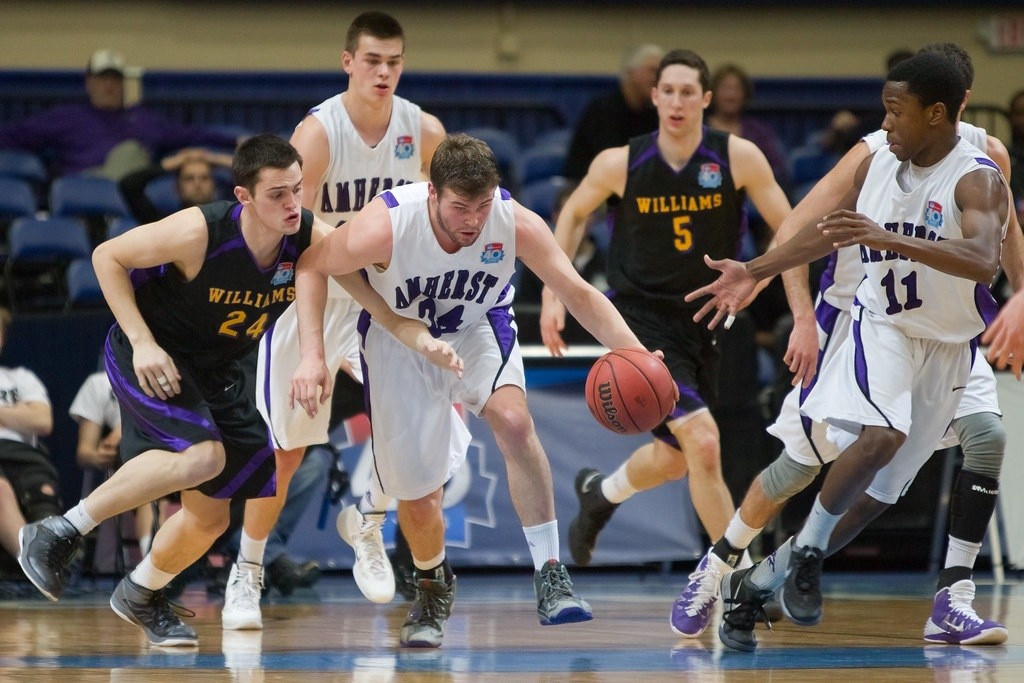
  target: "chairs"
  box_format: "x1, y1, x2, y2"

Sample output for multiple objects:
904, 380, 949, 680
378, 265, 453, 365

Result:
0, 105, 884, 594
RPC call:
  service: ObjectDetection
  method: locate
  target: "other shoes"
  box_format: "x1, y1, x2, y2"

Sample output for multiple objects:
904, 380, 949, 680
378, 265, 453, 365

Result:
261, 556, 322, 598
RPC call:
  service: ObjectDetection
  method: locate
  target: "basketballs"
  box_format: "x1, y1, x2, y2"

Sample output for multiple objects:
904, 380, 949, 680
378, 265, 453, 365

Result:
585, 348, 674, 435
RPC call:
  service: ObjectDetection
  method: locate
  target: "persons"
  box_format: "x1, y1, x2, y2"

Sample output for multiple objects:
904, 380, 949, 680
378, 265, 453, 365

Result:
1, 44, 1024, 651
221, 12, 445, 632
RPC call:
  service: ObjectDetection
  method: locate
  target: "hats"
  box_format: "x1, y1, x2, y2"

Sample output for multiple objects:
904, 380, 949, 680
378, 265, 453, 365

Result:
89, 49, 126, 76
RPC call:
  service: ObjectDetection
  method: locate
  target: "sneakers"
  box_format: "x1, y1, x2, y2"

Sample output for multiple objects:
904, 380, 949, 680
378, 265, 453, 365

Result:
400, 572, 458, 648
779, 531, 824, 625
15, 514, 85, 603
534, 559, 594, 625
922, 579, 1010, 646
670, 545, 735, 639
390, 552, 417, 601
110, 571, 200, 647
335, 504, 397, 604
568, 467, 624, 566
718, 560, 783, 652
221, 561, 265, 631
